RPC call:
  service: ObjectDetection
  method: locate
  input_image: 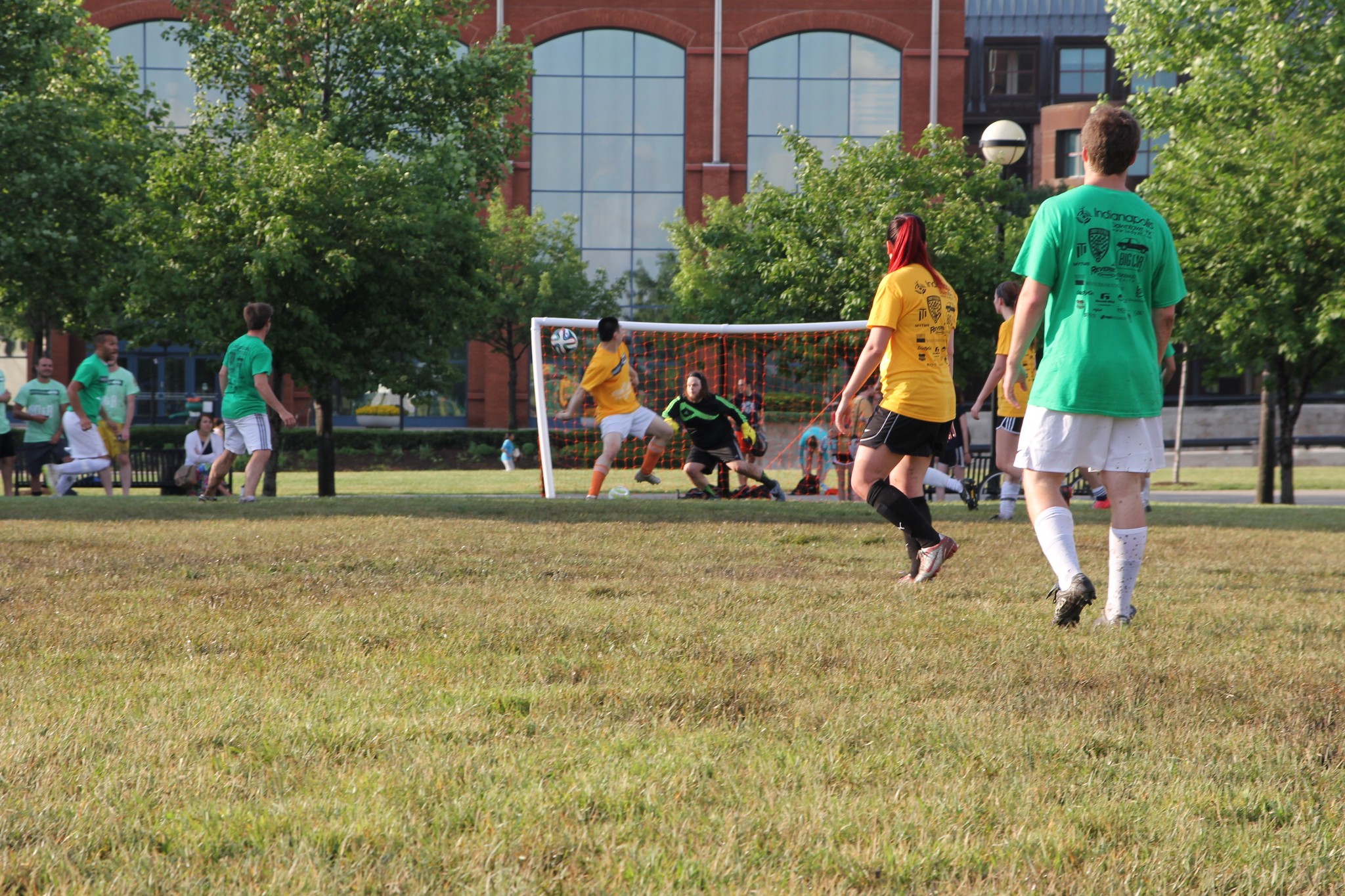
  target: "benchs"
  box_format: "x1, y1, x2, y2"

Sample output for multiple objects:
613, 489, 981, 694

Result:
14, 449, 233, 497
929, 445, 1092, 494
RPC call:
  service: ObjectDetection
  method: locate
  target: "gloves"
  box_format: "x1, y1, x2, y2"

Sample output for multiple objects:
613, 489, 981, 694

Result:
663, 418, 680, 433
741, 421, 757, 446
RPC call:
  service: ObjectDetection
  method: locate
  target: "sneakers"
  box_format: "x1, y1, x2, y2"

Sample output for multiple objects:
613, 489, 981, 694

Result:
960, 479, 979, 510
1046, 573, 1096, 628
990, 514, 1013, 522
897, 573, 915, 586
770, 479, 785, 502
1093, 497, 1111, 509
634, 471, 662, 485
912, 533, 959, 584
586, 496, 597, 501
1061, 485, 1073, 506
1095, 605, 1137, 629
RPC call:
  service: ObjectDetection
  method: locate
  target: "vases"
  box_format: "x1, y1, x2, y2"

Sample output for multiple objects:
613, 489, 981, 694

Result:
356, 414, 400, 427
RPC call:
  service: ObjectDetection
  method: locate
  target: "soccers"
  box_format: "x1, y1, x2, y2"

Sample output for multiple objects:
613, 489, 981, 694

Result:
551, 328, 578, 356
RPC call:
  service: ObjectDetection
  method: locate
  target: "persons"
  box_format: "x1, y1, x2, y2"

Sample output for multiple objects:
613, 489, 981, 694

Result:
501, 433, 517, 471
96, 353, 141, 496
42, 330, 118, 496
13, 356, 77, 496
661, 372, 785, 502
836, 214, 959, 585
184, 413, 233, 497
728, 377, 764, 492
1004, 106, 1190, 627
200, 303, 296, 503
0, 368, 16, 496
213, 417, 224, 439
555, 316, 672, 500
240, 484, 245, 496
799, 281, 1176, 523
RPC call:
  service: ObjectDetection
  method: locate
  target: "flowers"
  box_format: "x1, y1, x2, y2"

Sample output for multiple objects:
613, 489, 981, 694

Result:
356, 405, 401, 415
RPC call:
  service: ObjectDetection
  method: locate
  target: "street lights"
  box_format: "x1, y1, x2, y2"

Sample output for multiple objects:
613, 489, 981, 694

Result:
978, 118, 1028, 494
